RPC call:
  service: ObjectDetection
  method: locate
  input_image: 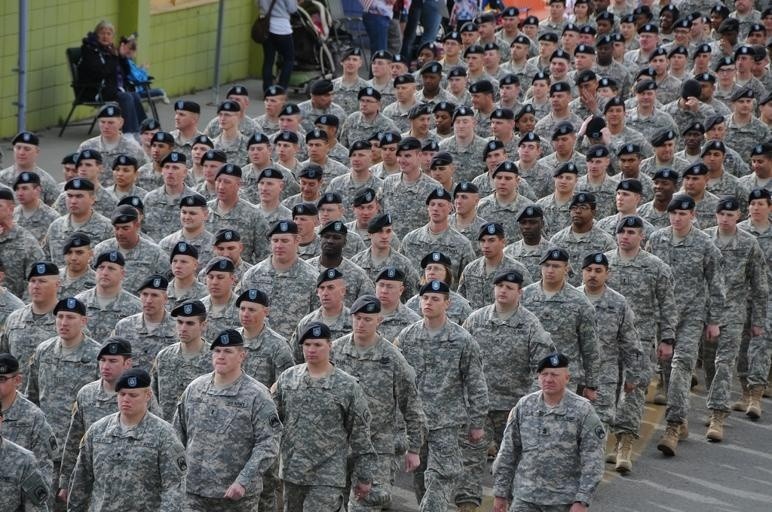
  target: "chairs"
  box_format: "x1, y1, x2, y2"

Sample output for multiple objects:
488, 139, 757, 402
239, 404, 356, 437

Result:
323, 1, 369, 70
58, 47, 162, 136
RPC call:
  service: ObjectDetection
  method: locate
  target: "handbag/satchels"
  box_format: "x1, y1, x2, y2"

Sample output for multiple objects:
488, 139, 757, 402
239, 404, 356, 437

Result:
250, 11, 274, 43
74, 44, 108, 98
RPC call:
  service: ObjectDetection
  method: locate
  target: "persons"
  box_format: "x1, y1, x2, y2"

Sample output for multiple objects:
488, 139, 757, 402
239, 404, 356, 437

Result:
1, 2, 771, 512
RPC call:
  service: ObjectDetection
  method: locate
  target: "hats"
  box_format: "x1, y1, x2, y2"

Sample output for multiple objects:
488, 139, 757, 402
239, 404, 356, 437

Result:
117, 196, 143, 211
615, 217, 643, 233
517, 205, 542, 222
715, 195, 739, 214
748, 188, 769, 204
349, 295, 382, 315
26, 262, 59, 282
342, 0, 772, 76
235, 289, 269, 308
426, 188, 451, 206
568, 193, 596, 212
62, 232, 90, 254
210, 330, 243, 350
0, 187, 14, 200
211, 229, 241, 246
536, 353, 569, 375
53, 297, 86, 315
539, 248, 568, 264
453, 182, 479, 199
11, 130, 38, 146
268, 187, 392, 236
13, 171, 40, 190
139, 274, 168, 290
419, 281, 450, 295
116, 370, 149, 393
375, 267, 406, 282
315, 267, 344, 288
666, 196, 695, 211
475, 222, 504, 241
205, 255, 235, 276
420, 252, 451, 269
170, 241, 199, 263
61, 149, 138, 191
93, 251, 125, 269
299, 322, 331, 344
0, 352, 19, 374
95, 75, 772, 192
97, 340, 133, 361
171, 300, 206, 317
492, 269, 524, 282
178, 193, 207, 208
581, 253, 609, 270
111, 204, 138, 225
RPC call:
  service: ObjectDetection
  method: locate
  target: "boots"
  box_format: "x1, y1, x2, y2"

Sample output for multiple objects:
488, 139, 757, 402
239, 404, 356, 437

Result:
731, 399, 745, 411
745, 385, 765, 422
615, 434, 634, 473
653, 373, 667, 405
657, 422, 681, 457
606, 452, 616, 463
707, 410, 726, 443
682, 417, 690, 440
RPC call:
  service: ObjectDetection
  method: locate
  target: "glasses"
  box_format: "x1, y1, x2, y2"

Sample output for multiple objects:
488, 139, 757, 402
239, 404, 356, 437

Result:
571, 204, 591, 211
0, 374, 18, 383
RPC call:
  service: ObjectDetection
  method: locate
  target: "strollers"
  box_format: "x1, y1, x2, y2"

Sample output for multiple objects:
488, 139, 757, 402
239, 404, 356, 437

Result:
314, 15, 364, 75
